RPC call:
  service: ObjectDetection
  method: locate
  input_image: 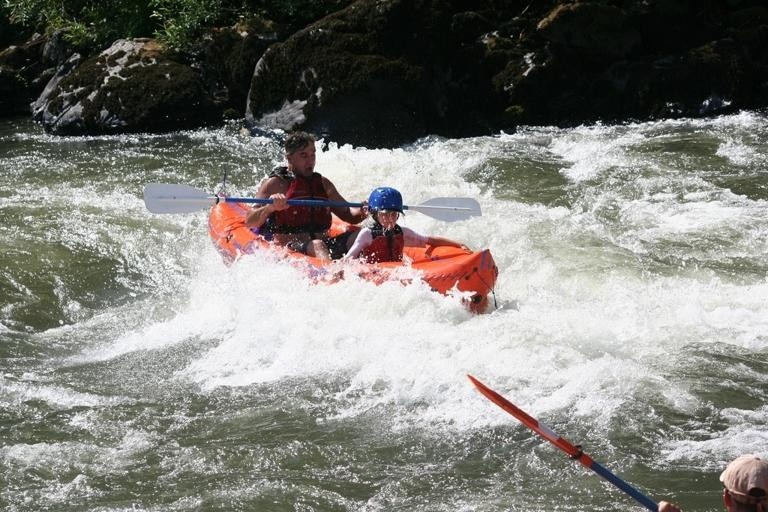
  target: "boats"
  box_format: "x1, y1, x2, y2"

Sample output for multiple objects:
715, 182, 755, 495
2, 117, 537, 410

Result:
205, 190, 501, 317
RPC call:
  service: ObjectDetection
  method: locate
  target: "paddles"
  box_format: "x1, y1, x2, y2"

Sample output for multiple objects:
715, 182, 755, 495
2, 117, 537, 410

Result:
468, 376, 658, 512
145, 184, 482, 222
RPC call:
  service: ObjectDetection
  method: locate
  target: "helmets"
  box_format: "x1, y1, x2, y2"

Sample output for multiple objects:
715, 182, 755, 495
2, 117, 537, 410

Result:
369, 187, 407, 217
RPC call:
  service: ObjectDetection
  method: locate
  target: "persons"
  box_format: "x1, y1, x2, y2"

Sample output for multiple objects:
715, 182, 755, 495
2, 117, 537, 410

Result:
245, 130, 370, 261
656, 453, 768, 512
340, 186, 472, 262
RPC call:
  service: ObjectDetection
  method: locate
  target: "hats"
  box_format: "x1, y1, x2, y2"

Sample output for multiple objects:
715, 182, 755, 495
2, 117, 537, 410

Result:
719, 455, 768, 498
